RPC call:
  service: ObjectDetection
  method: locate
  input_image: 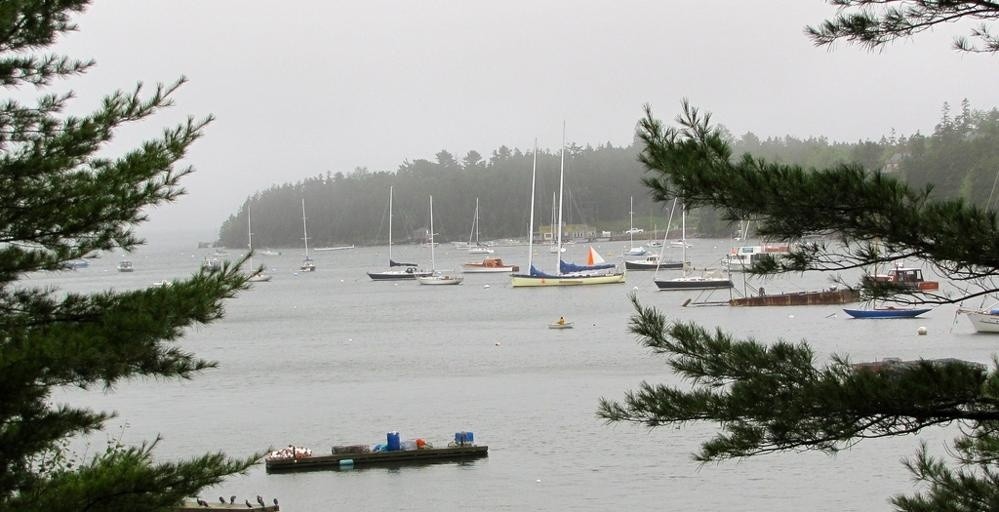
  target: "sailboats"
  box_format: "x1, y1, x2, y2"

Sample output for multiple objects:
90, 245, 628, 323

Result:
298, 197, 319, 273
198, 206, 279, 285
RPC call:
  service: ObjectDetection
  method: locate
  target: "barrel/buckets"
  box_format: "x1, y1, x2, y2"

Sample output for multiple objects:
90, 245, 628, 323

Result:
455, 431, 474, 441
386, 431, 399, 450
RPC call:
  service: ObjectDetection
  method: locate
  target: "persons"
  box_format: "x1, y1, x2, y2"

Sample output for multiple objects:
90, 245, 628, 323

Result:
560, 317, 564, 325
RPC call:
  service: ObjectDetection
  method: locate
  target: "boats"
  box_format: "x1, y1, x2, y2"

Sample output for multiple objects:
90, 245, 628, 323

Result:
311, 243, 356, 253
152, 279, 173, 288
860, 264, 939, 288
958, 304, 999, 336
262, 438, 488, 473
111, 257, 135, 275
548, 320, 573, 329
841, 304, 936, 318
